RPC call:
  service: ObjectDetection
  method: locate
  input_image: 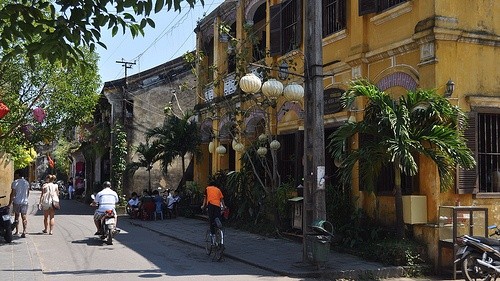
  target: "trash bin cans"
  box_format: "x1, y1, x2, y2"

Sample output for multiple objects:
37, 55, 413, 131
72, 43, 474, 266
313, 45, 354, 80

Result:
304, 219, 334, 269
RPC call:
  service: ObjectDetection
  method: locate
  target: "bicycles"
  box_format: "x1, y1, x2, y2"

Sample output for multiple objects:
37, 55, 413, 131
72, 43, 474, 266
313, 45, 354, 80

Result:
200, 205, 229, 263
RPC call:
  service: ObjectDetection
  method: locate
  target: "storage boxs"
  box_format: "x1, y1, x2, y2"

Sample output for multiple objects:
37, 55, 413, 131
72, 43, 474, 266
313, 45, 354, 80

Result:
394, 195, 428, 225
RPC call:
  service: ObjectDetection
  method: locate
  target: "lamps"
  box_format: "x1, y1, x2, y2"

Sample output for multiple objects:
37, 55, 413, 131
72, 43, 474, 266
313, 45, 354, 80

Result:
444, 77, 455, 98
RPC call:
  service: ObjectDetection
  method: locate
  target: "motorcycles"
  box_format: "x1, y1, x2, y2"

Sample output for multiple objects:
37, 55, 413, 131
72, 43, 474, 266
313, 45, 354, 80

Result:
0, 195, 20, 244
89, 194, 121, 245
452, 225, 500, 281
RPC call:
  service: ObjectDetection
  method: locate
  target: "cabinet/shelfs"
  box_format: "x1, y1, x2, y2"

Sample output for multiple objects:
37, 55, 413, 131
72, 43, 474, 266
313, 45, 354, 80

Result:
438, 205, 488, 280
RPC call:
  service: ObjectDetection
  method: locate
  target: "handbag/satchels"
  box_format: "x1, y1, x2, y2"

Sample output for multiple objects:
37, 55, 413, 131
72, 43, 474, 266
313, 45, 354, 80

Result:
52, 201, 60, 209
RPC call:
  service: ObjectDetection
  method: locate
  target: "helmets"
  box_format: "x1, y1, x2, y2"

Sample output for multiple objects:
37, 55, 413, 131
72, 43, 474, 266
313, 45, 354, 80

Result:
102, 181, 111, 187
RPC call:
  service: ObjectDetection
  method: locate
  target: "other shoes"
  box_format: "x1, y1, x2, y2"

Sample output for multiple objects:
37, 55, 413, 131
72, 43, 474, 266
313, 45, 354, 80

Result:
49, 232, 52, 234
21, 233, 26, 238
42, 230, 47, 234
95, 231, 102, 235
13, 233, 19, 235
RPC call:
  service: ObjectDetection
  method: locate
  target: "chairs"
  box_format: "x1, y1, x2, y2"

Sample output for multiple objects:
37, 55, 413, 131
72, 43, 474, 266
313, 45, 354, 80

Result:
164, 202, 177, 220
154, 202, 164, 222
